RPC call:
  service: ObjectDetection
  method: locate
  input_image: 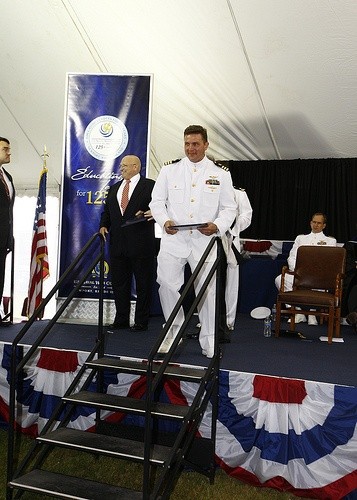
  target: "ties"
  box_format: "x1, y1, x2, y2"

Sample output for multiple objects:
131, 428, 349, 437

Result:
0, 168, 10, 200
121, 179, 131, 215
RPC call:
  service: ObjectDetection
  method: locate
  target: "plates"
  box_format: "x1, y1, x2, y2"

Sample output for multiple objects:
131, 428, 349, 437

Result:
250, 307, 271, 319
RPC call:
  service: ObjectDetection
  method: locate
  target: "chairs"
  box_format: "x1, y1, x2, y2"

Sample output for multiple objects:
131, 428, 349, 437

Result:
275, 245, 346, 344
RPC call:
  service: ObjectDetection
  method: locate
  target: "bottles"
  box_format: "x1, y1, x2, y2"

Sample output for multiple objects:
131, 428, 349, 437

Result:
264, 316, 271, 337
272, 304, 276, 321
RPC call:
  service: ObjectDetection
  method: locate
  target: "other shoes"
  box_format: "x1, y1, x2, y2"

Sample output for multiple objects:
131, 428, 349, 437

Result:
308, 314, 318, 325
130, 324, 148, 331
288, 314, 306, 323
108, 323, 129, 330
202, 349, 222, 359
157, 337, 183, 353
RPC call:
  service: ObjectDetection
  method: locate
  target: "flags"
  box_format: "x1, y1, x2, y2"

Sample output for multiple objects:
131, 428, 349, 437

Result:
26, 166, 50, 323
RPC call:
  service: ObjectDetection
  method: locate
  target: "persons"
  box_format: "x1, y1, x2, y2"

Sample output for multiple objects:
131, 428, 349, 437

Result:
273, 209, 338, 325
0, 137, 16, 326
146, 124, 237, 360
224, 185, 253, 329
99, 155, 157, 333
